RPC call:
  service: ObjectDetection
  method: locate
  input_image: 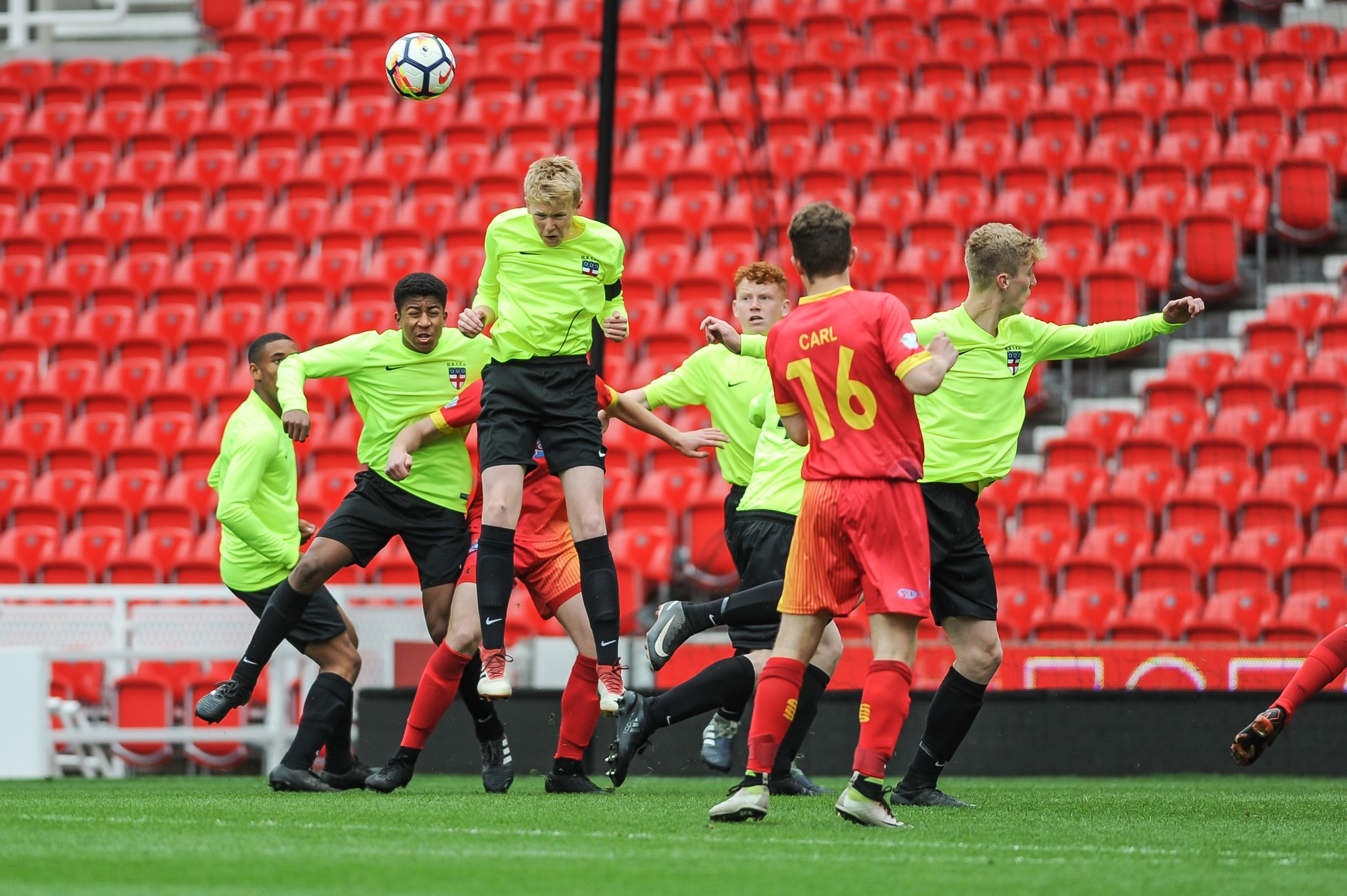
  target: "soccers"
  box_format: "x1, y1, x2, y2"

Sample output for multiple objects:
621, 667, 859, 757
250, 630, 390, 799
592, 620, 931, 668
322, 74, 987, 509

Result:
386, 33, 456, 100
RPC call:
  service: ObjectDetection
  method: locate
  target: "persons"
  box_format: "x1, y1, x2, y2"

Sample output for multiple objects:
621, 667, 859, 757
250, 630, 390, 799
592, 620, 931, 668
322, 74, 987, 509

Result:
603, 385, 824, 797
456, 155, 630, 713
363, 353, 732, 797
207, 331, 376, 791
700, 222, 1205, 810
1230, 623, 1347, 766
705, 201, 959, 828
597, 261, 831, 792
192, 272, 513, 793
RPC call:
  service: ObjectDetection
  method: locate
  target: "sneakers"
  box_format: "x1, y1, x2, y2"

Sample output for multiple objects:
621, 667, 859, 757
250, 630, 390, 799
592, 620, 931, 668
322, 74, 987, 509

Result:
700, 712, 741, 773
770, 771, 821, 797
605, 691, 655, 788
544, 771, 611, 795
194, 673, 252, 725
708, 781, 769, 821
1230, 707, 1283, 768
890, 781, 977, 808
319, 754, 373, 792
835, 771, 914, 829
477, 646, 513, 700
596, 657, 630, 713
364, 754, 416, 794
269, 762, 341, 792
644, 600, 694, 672
478, 733, 513, 793
789, 752, 832, 794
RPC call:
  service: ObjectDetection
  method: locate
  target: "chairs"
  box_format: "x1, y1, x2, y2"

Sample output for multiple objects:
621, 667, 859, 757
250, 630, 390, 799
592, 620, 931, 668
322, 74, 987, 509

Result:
0, 0, 1347, 778
